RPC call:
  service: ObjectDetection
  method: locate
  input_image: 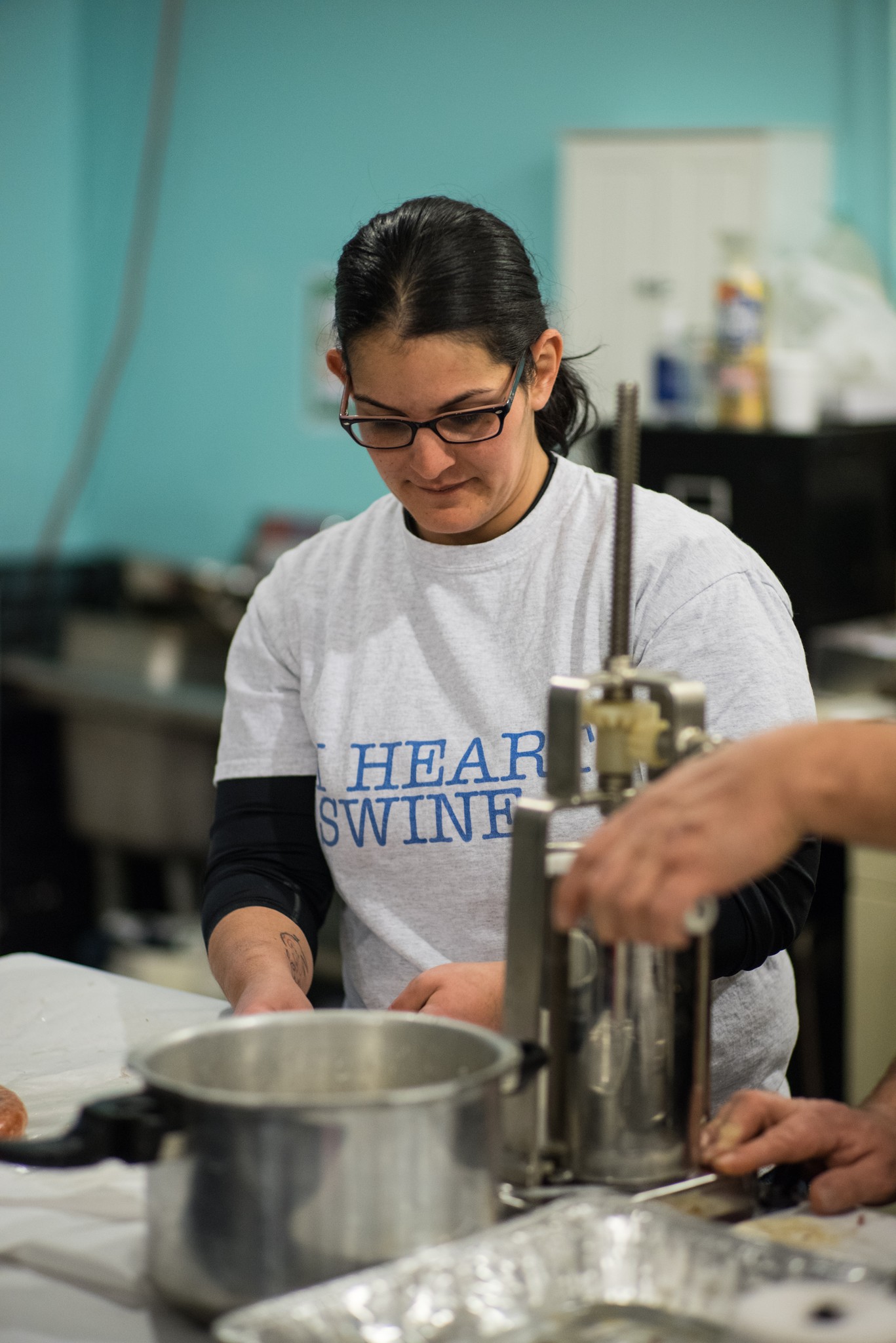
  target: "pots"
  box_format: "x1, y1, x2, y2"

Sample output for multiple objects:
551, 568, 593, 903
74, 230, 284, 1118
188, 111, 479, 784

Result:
0, 1010, 556, 1324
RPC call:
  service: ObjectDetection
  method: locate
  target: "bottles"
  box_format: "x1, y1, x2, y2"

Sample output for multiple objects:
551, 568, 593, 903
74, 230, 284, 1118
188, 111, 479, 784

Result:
712, 232, 772, 436
652, 290, 698, 427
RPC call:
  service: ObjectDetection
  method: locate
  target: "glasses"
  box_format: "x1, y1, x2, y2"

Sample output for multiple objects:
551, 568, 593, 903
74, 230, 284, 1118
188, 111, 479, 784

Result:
337, 352, 526, 453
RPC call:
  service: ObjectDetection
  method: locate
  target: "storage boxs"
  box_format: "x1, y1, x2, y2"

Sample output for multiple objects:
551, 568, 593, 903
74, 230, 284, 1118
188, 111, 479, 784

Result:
216, 1193, 895, 1343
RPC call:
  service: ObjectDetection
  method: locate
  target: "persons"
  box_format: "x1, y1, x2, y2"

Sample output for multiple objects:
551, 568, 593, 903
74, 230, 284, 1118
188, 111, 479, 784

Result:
537, 656, 892, 1217
194, 193, 840, 1214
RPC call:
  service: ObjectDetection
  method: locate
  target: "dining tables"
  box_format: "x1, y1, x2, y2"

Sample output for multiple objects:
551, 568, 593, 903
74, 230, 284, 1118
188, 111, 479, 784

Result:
0, 949, 896, 1341
